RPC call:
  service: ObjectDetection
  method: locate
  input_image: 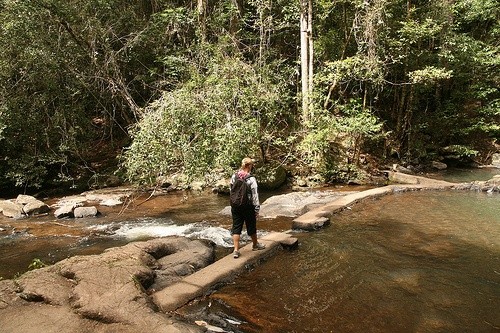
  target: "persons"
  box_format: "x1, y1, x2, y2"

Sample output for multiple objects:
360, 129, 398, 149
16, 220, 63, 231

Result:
230, 158, 266, 258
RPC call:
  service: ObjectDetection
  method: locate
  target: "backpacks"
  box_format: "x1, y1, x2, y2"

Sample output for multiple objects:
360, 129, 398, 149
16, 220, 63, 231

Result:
230, 171, 256, 209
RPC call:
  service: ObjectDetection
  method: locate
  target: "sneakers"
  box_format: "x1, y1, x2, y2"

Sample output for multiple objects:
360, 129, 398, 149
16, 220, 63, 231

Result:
232, 250, 241, 258
252, 242, 267, 251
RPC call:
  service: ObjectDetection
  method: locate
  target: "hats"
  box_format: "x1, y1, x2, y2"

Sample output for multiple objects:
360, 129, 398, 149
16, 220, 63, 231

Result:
241, 157, 256, 166
